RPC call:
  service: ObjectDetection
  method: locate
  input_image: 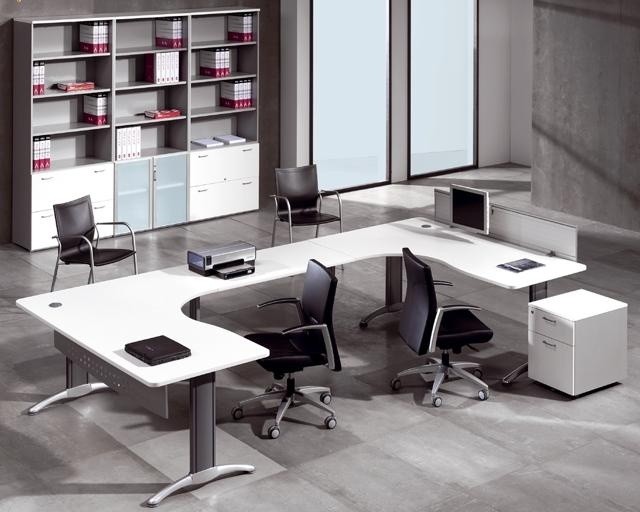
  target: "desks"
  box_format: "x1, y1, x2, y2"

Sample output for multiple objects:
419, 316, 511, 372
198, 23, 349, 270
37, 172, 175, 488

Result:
15, 217, 587, 508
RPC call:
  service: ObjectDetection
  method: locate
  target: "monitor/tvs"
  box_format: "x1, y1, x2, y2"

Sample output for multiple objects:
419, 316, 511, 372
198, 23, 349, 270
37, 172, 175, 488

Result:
448, 182, 491, 237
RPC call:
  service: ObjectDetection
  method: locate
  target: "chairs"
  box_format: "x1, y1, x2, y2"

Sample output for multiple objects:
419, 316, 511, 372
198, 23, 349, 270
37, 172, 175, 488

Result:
231, 259, 342, 439
269, 164, 343, 247
50, 195, 138, 292
390, 247, 494, 406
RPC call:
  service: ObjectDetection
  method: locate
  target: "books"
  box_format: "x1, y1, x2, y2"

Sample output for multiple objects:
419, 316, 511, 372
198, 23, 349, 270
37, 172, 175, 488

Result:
116, 127, 142, 159
143, 52, 179, 83
192, 136, 224, 148
83, 94, 108, 126
214, 134, 246, 144
56, 81, 95, 92
227, 13, 253, 42
33, 62, 45, 94
220, 80, 253, 109
77, 21, 109, 53
155, 17, 184, 47
199, 48, 231, 77
33, 137, 50, 172
145, 108, 181, 119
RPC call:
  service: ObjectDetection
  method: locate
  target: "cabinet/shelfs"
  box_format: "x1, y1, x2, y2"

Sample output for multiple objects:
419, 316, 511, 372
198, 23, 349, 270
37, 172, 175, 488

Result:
97, 9, 190, 238
528, 288, 628, 399
173, 5, 260, 226
11, 16, 115, 251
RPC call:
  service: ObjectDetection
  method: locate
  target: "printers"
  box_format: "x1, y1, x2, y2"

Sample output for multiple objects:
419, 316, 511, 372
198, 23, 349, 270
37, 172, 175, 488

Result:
186, 239, 258, 281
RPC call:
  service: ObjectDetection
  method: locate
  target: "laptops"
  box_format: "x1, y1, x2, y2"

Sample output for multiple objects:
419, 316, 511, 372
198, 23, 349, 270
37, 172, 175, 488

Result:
124, 334, 191, 367
